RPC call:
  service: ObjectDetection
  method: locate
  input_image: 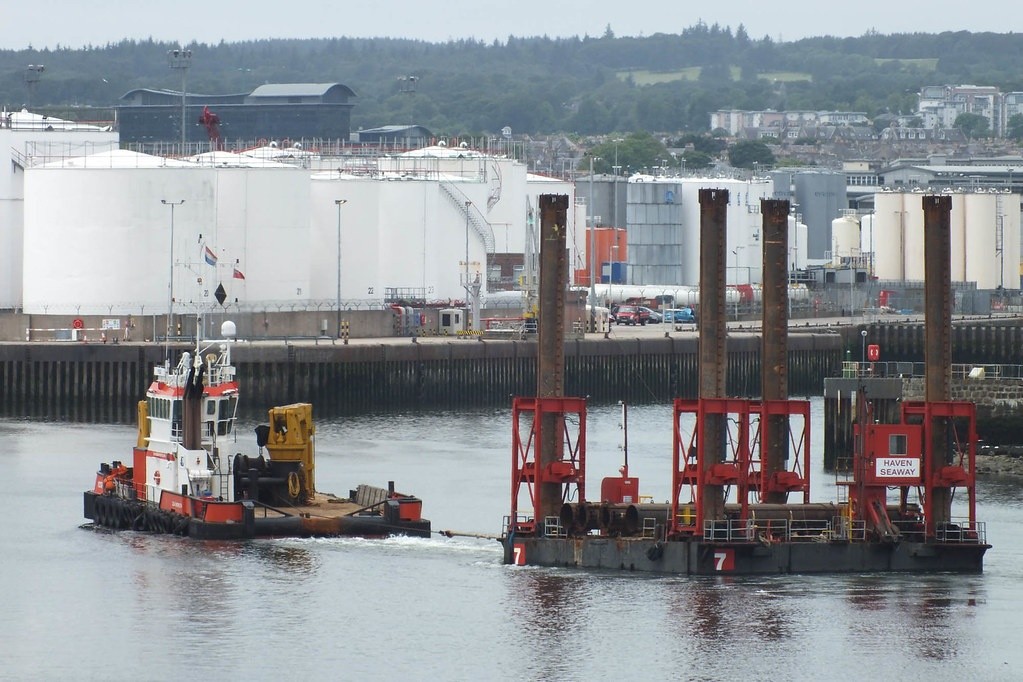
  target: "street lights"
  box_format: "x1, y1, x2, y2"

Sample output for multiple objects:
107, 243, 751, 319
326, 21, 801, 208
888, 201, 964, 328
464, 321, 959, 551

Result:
608, 246, 619, 314
894, 210, 908, 281
161, 199, 185, 335
612, 138, 623, 259
464, 201, 472, 333
335, 199, 346, 338
397, 76, 419, 158
792, 204, 800, 285
26, 65, 44, 127
165, 50, 192, 156
733, 246, 744, 321
584, 152, 602, 333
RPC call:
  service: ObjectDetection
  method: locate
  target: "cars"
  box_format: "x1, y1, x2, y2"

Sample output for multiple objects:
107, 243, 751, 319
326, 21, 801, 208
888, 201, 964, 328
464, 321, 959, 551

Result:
612, 305, 662, 324
664, 309, 694, 322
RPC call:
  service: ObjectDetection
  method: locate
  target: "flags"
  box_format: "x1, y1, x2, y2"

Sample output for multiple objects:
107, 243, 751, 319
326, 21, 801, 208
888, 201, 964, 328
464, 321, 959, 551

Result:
233, 268, 245, 280
204, 246, 218, 267
214, 283, 227, 305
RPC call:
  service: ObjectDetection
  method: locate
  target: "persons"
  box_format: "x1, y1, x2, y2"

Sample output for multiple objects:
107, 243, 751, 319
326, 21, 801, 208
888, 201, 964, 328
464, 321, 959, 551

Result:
116, 461, 129, 478
103, 469, 117, 496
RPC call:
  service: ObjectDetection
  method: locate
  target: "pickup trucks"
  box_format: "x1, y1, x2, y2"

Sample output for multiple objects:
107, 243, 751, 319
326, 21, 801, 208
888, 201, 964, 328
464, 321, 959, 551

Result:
616, 306, 651, 325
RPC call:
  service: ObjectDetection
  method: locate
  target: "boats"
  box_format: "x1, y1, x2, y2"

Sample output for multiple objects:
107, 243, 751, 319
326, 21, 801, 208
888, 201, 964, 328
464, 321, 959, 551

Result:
496, 330, 992, 573
84, 234, 432, 538
